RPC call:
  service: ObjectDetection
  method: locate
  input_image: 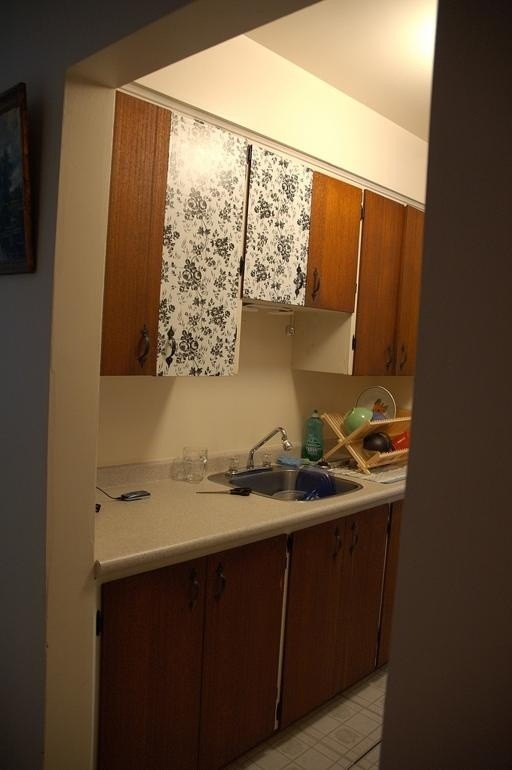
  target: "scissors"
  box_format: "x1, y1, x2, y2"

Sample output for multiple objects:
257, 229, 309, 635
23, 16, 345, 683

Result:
196, 487, 252, 496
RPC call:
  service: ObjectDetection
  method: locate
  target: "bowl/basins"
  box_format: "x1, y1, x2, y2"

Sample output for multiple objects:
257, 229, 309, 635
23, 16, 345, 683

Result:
362, 432, 391, 449
343, 407, 373, 436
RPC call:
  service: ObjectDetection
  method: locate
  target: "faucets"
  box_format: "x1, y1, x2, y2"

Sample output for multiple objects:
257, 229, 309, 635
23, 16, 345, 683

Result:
248, 426, 294, 470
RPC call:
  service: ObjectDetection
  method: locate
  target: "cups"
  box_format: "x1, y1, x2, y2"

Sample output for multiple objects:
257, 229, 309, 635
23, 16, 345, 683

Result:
183, 446, 209, 484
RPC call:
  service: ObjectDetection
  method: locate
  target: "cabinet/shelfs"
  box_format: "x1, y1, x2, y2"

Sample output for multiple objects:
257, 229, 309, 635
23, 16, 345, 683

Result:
280, 505, 388, 735
355, 188, 424, 376
240, 143, 359, 317
378, 498, 406, 667
98, 91, 171, 378
154, 113, 246, 378
103, 534, 290, 770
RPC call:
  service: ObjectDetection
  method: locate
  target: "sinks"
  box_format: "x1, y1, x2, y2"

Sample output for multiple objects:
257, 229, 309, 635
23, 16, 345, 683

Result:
207, 466, 363, 502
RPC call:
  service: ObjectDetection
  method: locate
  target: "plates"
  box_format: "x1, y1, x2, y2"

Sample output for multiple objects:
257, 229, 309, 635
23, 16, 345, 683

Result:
355, 385, 397, 432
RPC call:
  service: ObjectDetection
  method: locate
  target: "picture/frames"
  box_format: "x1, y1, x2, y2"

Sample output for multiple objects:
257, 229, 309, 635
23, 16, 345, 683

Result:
0, 77, 37, 274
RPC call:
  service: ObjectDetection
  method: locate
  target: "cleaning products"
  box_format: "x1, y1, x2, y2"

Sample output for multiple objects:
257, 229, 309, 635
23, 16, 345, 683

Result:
302, 410, 324, 462
